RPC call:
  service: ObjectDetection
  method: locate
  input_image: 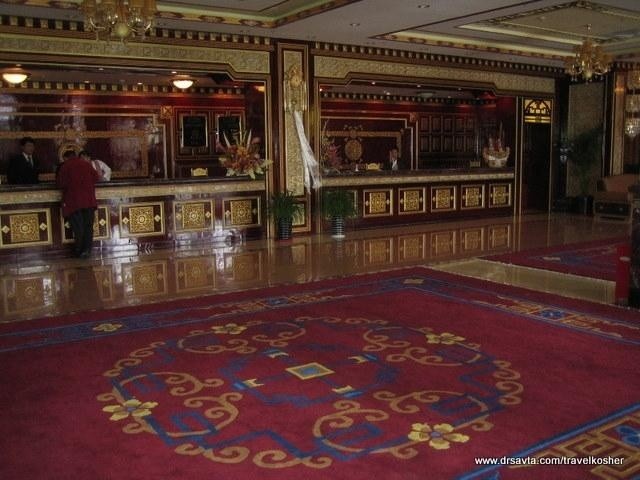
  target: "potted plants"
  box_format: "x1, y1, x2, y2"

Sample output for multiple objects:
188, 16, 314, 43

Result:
264, 189, 304, 239
562, 126, 604, 217
320, 185, 357, 239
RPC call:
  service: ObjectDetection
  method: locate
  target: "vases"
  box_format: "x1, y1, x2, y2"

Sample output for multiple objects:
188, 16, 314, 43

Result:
484, 147, 510, 168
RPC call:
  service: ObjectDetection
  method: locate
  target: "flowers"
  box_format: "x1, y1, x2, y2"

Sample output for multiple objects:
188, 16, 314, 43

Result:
213, 129, 273, 180
321, 121, 343, 176
483, 137, 510, 160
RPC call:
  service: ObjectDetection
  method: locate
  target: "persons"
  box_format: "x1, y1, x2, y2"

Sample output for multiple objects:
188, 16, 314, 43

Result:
55, 150, 102, 259
6, 136, 41, 185
78, 150, 113, 182
383, 147, 408, 170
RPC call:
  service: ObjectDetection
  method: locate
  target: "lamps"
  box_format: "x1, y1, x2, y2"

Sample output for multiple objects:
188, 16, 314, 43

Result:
560, 24, 617, 87
623, 52, 639, 141
167, 74, 198, 90
80, 1, 161, 47
1, 68, 30, 84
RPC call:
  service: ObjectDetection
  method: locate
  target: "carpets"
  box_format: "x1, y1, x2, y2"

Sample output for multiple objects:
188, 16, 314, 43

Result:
0, 235, 640, 476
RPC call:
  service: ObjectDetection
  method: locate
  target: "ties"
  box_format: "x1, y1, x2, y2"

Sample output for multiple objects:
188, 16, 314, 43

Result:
28, 155, 32, 167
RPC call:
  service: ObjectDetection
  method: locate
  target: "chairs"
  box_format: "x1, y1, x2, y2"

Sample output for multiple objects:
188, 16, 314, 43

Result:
591, 173, 639, 222
